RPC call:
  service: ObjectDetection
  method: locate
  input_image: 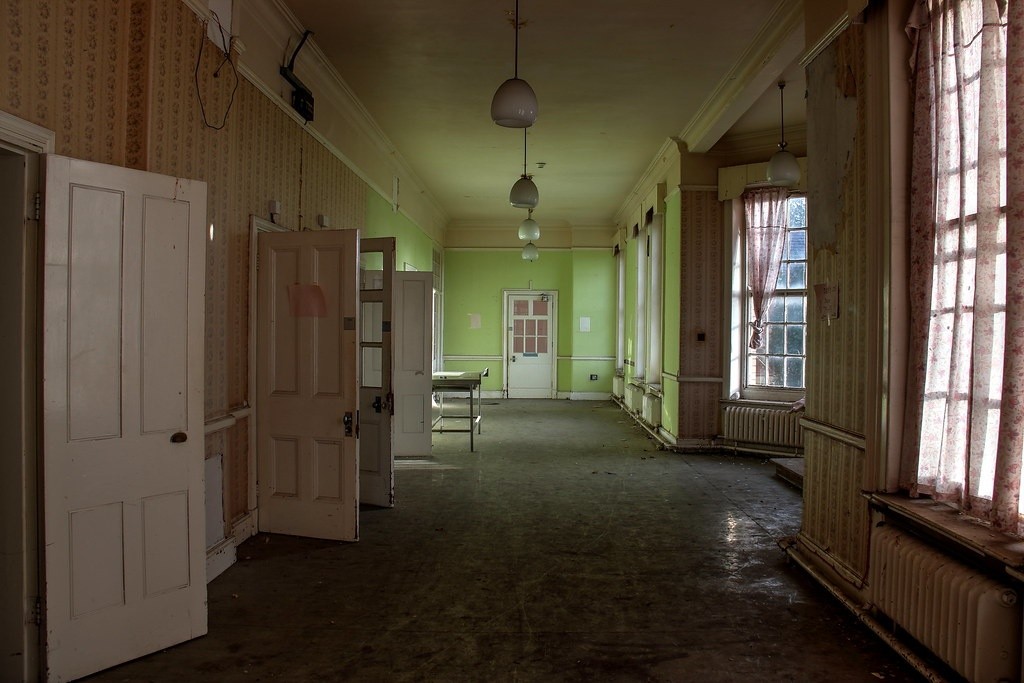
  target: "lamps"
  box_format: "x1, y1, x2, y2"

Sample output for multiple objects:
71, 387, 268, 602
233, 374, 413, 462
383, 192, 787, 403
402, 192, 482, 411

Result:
507, 124, 539, 209
490, 0, 540, 130
521, 240, 540, 260
517, 210, 542, 240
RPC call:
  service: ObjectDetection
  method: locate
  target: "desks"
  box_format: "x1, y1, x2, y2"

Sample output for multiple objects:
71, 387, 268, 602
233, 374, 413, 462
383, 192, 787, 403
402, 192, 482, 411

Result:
432, 368, 484, 454
